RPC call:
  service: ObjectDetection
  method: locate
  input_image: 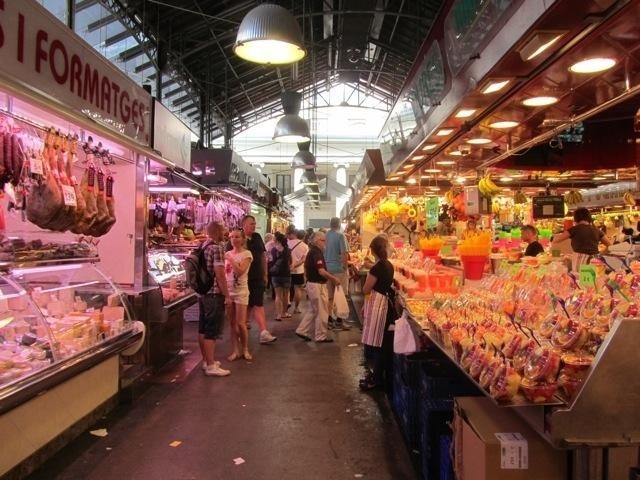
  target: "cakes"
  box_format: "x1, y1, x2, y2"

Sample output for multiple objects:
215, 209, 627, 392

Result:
424, 255, 640, 404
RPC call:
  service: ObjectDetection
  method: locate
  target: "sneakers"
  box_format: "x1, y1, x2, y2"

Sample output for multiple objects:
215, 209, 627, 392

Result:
296, 332, 311, 342
359, 375, 380, 390
228, 353, 241, 362
243, 351, 251, 360
260, 332, 277, 344
317, 338, 334, 343
206, 366, 230, 376
328, 320, 352, 330
275, 304, 302, 320
202, 360, 221, 369
246, 321, 252, 330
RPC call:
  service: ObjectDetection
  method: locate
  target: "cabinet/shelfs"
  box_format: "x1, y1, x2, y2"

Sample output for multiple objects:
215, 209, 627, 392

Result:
144, 249, 198, 367
0, 255, 143, 480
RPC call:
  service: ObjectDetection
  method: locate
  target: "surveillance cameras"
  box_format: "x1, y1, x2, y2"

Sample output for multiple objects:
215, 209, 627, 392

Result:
550, 139, 559, 148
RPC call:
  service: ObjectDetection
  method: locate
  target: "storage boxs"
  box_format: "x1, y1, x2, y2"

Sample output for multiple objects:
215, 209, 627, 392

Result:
452, 397, 566, 480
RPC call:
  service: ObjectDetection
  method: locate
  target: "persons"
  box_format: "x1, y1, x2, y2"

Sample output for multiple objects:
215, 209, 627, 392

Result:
612, 221, 640, 246
516, 225, 545, 258
552, 205, 613, 257
359, 232, 399, 392
404, 216, 484, 251
183, 212, 354, 385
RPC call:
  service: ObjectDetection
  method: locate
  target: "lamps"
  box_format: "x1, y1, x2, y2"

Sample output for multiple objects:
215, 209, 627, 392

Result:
232, 0, 320, 211
479, 30, 569, 93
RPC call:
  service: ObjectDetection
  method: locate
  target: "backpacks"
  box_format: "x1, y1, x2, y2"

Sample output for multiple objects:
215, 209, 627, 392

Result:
185, 242, 218, 295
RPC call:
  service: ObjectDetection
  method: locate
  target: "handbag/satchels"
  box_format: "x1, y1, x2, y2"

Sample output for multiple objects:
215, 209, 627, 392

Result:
268, 257, 286, 276
